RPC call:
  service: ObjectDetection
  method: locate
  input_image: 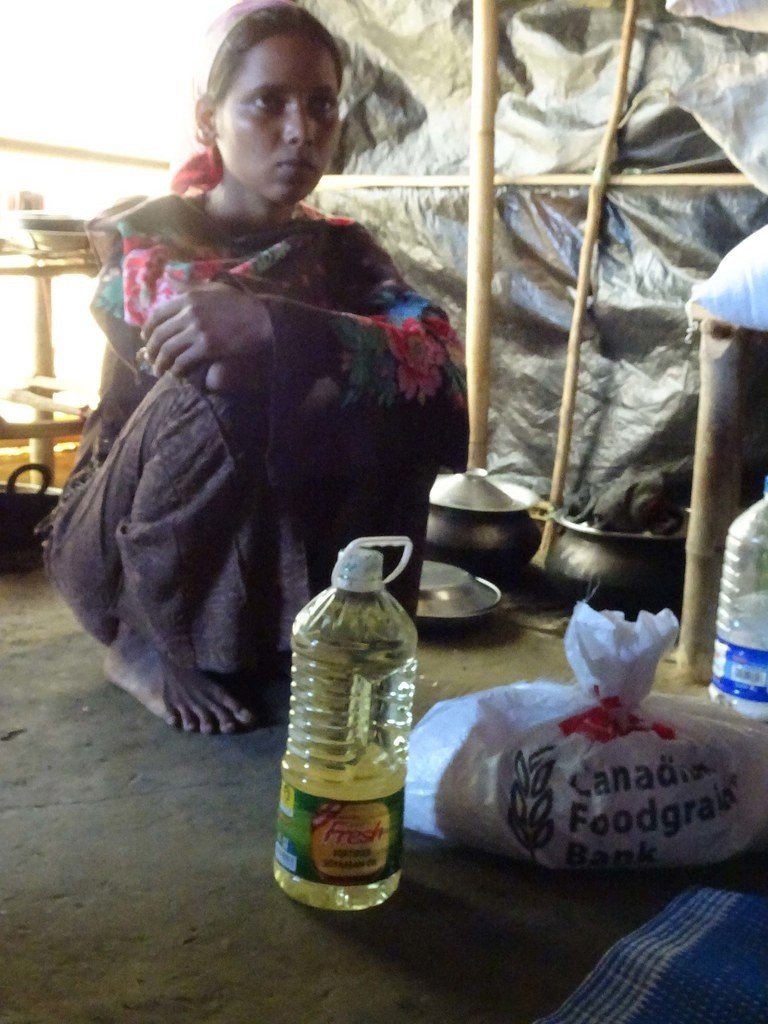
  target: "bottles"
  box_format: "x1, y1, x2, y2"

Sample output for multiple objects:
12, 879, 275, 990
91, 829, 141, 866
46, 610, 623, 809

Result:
273, 537, 418, 911
709, 476, 768, 723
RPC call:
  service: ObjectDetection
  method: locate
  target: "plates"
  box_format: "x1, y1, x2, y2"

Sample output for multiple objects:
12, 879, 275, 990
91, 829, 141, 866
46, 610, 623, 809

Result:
417, 559, 502, 617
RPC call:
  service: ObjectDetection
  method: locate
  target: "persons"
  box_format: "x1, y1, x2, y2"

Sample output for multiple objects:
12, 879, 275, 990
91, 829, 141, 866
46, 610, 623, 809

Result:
45, 0, 471, 734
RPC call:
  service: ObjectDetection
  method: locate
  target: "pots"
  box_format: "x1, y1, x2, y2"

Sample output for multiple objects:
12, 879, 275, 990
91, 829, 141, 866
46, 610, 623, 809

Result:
547, 509, 690, 613
0, 465, 63, 566
425, 468, 542, 577
